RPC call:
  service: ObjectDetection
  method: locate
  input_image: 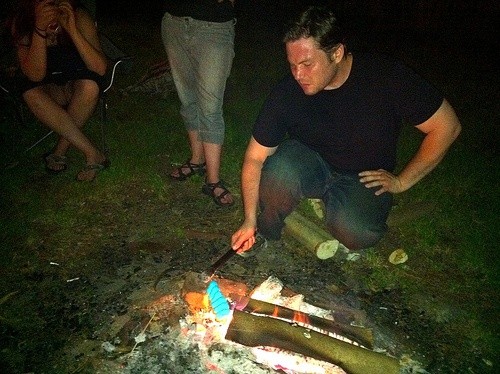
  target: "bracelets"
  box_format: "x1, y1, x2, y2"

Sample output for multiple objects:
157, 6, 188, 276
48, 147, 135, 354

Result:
34, 24, 47, 32
33, 28, 48, 39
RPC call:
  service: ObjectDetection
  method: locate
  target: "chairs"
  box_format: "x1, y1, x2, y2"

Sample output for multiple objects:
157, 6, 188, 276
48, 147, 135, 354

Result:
0, 0, 121, 170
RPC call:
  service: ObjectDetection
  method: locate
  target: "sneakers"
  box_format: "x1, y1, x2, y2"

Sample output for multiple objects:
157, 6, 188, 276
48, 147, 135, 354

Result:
236, 234, 267, 258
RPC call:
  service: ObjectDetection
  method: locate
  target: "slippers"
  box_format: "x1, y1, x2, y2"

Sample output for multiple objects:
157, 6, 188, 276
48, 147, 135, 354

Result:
47, 154, 66, 173
76, 160, 110, 183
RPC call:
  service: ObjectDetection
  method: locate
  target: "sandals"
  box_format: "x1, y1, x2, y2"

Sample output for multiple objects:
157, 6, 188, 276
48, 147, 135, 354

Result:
203, 180, 236, 207
170, 160, 206, 181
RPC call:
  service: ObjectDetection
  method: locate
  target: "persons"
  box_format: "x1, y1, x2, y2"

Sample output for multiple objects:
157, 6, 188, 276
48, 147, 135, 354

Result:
160, 0, 236, 206
11, 0, 111, 183
230, 5, 461, 254
